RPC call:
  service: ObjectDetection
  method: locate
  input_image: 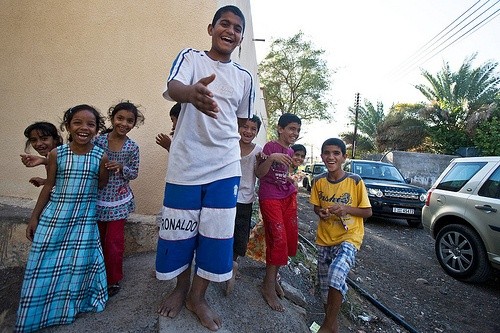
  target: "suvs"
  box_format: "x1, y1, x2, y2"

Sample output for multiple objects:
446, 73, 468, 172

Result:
342, 160, 428, 229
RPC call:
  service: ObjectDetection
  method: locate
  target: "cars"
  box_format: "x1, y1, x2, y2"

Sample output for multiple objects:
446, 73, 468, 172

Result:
422, 156, 500, 284
306, 163, 329, 191
302, 164, 312, 187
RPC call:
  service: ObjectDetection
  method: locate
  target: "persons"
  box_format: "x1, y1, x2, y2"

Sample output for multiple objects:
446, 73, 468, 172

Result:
310, 138, 372, 333
255, 113, 302, 312
16, 104, 110, 333
155, 5, 256, 331
91, 100, 145, 297
245, 144, 306, 283
20, 121, 64, 187
233, 114, 269, 262
155, 102, 238, 296
